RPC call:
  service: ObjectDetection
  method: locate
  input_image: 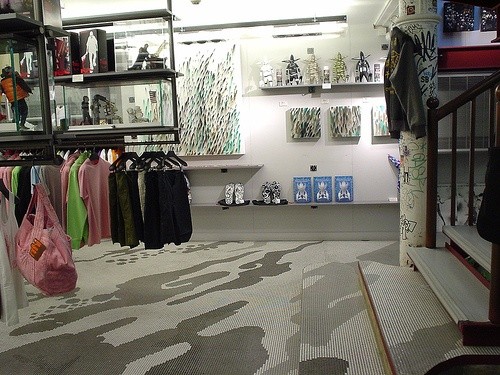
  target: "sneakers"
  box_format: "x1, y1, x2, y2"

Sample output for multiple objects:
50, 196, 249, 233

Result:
261, 181, 271, 204
226, 183, 234, 205
235, 181, 244, 204
270, 181, 281, 204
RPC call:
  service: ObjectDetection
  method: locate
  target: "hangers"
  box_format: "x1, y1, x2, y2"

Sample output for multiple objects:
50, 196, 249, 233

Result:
0, 141, 188, 205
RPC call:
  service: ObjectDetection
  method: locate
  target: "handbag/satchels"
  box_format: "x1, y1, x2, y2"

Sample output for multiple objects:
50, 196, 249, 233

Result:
15, 183, 78, 295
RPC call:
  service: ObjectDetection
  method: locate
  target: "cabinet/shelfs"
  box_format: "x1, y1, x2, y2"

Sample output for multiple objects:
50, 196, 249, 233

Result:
21, 1, 184, 134
0, 0, 70, 142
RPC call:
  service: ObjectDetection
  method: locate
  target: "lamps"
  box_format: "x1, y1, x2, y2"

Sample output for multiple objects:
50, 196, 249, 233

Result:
272, 21, 348, 38
177, 29, 226, 45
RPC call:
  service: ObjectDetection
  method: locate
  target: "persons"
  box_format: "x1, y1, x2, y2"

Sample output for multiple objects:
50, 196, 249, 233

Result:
80, 96, 93, 125
0, 66, 34, 130
129, 43, 150, 70
